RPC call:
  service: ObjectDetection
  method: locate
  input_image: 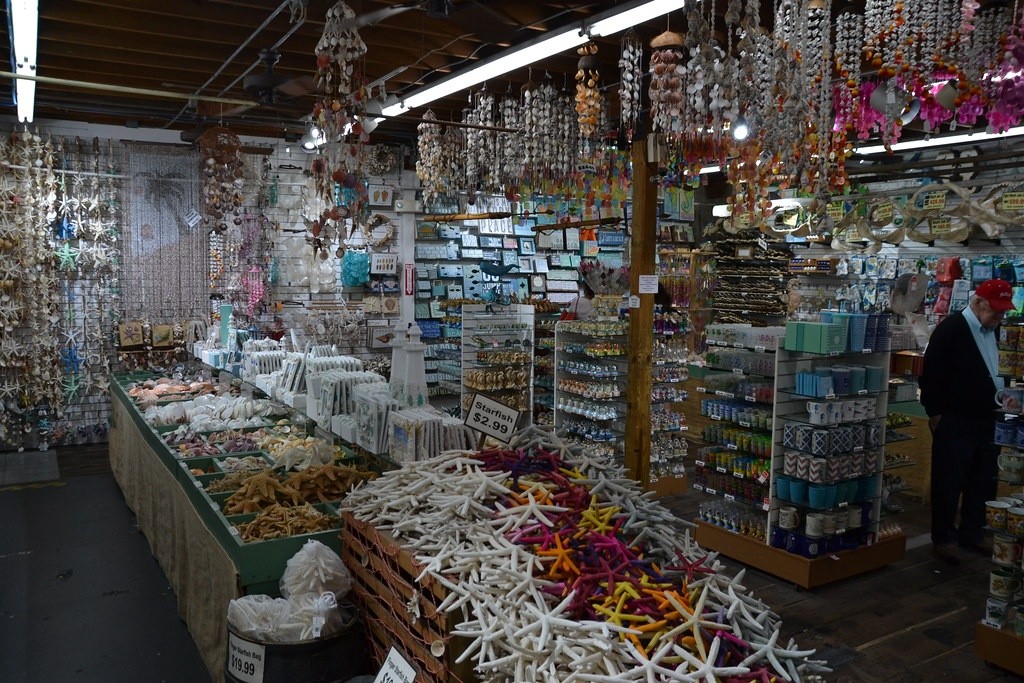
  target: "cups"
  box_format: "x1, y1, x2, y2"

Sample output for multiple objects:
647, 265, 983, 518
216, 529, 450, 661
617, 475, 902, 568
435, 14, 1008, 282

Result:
984, 318, 1024, 638
776, 312, 890, 559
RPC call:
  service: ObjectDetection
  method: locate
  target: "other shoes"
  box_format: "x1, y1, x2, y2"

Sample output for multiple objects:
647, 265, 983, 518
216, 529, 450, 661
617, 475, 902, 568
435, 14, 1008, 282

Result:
975, 537, 994, 553
933, 542, 957, 556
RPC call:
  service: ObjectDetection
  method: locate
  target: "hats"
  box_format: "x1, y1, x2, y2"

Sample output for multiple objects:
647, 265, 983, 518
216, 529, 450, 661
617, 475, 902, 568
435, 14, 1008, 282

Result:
975, 279, 1017, 311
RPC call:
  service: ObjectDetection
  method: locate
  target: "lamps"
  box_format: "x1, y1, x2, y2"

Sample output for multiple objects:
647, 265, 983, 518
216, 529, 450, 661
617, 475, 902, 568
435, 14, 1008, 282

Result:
712, 197, 811, 217
304, 0, 685, 149
6, 0, 38, 122
683, 120, 1024, 176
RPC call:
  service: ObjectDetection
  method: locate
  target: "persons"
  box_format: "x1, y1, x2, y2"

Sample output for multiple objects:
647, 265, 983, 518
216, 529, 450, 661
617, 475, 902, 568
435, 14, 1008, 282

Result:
917, 279, 1016, 562
565, 278, 595, 320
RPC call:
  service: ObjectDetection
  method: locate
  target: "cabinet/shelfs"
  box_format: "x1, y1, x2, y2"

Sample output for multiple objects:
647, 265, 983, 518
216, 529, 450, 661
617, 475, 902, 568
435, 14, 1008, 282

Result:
553, 320, 693, 498
345, 515, 473, 683
716, 237, 838, 276
437, 303, 565, 433
692, 341, 919, 589
